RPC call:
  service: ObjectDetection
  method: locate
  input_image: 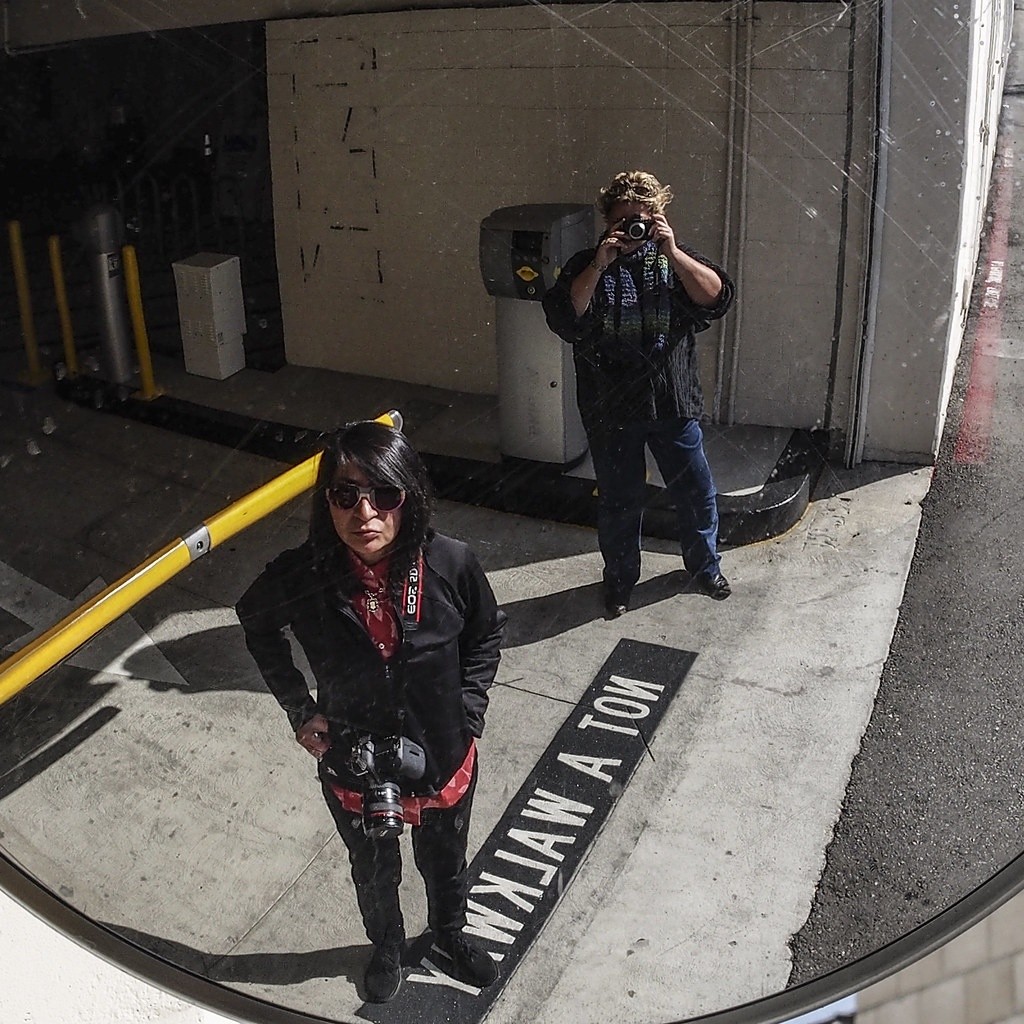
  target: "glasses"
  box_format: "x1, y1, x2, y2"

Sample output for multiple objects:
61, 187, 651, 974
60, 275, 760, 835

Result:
326, 482, 407, 512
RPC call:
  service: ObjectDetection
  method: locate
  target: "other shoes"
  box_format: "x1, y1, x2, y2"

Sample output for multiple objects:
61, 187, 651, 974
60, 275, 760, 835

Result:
606, 586, 631, 613
699, 571, 732, 600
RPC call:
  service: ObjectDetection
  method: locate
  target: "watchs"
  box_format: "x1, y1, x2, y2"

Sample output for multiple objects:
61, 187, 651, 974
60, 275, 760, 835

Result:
590, 259, 608, 272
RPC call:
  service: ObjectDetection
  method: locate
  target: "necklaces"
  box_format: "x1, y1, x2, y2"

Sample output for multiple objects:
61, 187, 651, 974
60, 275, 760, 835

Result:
361, 587, 385, 611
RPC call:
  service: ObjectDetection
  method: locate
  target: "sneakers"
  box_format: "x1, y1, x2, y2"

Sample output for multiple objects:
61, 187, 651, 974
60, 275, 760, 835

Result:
429, 929, 498, 986
364, 945, 402, 1001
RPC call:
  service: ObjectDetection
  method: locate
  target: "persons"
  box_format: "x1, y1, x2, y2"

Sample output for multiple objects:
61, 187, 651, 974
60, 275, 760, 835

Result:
235, 422, 508, 1000
542, 171, 738, 614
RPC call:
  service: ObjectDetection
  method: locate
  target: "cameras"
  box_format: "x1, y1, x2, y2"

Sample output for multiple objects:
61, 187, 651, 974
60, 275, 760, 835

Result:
624, 218, 656, 240
347, 735, 427, 844
